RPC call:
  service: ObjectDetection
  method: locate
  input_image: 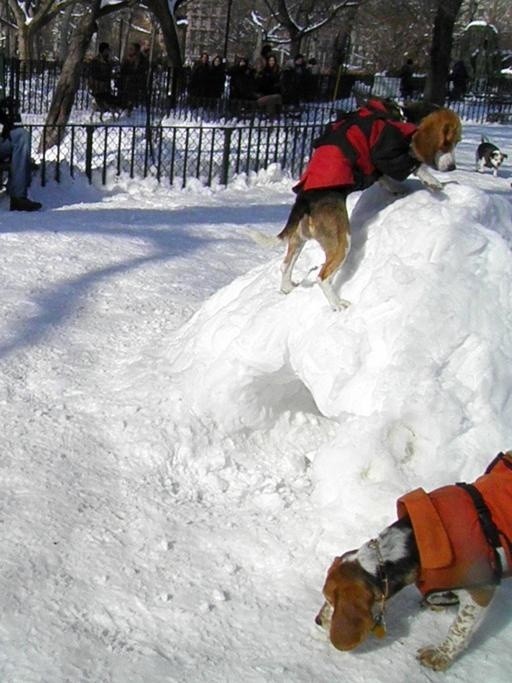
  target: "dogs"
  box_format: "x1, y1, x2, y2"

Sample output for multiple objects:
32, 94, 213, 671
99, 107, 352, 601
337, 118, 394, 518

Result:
313, 447, 512, 674
276, 97, 464, 313
474, 133, 508, 178
86, 90, 125, 122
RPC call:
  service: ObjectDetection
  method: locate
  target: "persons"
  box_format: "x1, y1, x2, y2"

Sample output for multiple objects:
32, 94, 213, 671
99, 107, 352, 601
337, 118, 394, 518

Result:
186, 45, 318, 99
0, 125, 42, 211
88, 37, 151, 97
399, 58, 418, 105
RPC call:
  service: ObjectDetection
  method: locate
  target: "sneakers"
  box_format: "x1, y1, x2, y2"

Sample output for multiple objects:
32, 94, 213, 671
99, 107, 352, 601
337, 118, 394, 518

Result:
9, 195, 43, 212
